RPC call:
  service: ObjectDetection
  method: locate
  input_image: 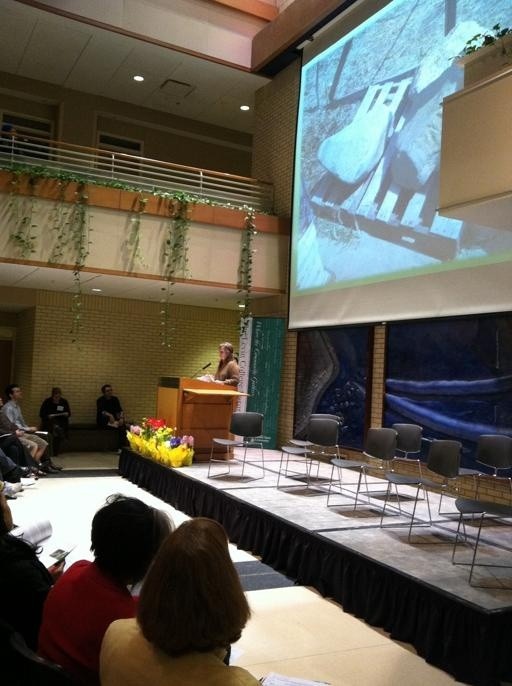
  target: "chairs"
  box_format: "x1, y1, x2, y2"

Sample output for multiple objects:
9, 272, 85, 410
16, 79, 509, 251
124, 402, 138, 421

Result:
6, 630, 69, 685
277, 414, 423, 518
380, 435, 512, 589
210, 412, 265, 483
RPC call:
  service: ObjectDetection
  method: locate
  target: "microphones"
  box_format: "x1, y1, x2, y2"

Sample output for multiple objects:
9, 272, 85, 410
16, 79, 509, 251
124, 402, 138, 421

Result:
191, 362, 211, 378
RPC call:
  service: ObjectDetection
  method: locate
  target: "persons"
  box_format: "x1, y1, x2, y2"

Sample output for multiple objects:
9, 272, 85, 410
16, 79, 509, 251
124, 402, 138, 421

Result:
37, 494, 176, 685
0, 492, 66, 652
215, 342, 240, 392
95, 384, 126, 455
98, 517, 263, 686
0, 384, 71, 500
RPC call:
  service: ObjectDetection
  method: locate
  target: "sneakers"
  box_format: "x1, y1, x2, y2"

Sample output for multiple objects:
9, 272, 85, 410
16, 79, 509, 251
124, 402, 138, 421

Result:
3, 460, 63, 495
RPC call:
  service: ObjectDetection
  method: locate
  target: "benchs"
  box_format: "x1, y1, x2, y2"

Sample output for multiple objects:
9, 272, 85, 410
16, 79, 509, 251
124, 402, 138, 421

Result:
51, 424, 125, 454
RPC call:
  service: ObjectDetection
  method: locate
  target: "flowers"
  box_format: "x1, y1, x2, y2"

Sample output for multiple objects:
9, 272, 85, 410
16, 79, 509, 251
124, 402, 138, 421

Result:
129, 416, 195, 449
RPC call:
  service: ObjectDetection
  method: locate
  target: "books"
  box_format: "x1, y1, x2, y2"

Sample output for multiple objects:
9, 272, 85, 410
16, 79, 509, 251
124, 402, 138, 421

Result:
8, 518, 79, 570
